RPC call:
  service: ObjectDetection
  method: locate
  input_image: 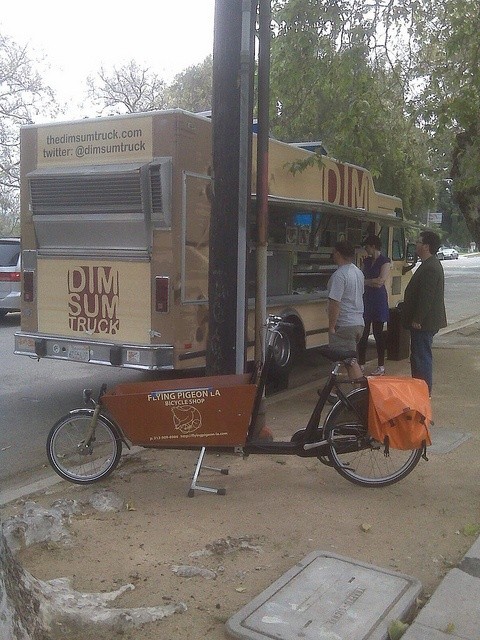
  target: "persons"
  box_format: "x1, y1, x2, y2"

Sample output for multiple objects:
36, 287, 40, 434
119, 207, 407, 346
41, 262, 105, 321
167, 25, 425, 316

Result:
356, 235, 390, 375
319, 242, 365, 406
401, 231, 447, 400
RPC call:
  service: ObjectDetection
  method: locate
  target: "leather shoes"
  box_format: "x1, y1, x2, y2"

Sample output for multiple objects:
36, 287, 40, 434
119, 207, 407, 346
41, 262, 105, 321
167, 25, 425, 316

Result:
317, 390, 337, 405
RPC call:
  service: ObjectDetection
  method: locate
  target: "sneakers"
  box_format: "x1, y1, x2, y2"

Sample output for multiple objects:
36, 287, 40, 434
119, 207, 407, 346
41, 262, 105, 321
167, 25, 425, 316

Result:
370, 367, 385, 376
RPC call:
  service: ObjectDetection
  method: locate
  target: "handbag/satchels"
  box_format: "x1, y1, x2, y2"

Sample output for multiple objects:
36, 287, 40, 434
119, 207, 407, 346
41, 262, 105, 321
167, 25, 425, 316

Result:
364, 376, 434, 461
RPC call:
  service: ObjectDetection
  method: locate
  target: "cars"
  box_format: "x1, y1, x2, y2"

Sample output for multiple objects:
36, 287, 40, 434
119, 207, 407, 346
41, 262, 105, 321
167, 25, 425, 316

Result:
436, 252, 445, 260
440, 248, 459, 260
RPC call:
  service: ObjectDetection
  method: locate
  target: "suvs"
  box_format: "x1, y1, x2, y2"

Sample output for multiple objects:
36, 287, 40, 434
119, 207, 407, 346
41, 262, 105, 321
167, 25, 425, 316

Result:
0, 237, 21, 320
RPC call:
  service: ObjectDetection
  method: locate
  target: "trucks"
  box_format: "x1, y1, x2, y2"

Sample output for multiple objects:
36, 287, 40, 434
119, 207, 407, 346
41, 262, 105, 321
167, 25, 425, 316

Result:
13, 107, 418, 374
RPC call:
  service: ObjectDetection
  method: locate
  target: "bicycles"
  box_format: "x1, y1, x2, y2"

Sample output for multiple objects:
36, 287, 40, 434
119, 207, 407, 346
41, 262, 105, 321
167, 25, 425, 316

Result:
46, 313, 432, 497
